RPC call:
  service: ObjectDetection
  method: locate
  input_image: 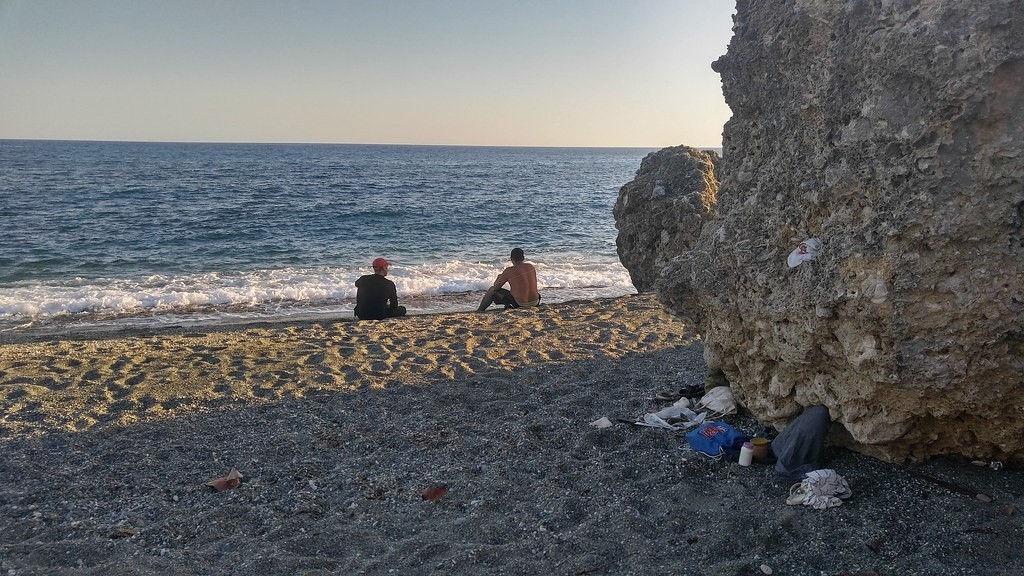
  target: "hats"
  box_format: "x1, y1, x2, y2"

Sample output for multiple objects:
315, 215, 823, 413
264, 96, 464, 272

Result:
373, 258, 392, 268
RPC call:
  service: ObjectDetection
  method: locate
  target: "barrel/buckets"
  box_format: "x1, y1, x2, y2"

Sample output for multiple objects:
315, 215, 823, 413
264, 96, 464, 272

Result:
751, 438, 769, 463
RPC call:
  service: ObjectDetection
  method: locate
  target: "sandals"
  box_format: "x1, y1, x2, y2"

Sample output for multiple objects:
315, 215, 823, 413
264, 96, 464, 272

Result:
678, 384, 706, 397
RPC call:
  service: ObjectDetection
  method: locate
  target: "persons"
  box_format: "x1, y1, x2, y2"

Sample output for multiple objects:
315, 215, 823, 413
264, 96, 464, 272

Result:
476, 248, 541, 311
354, 258, 407, 320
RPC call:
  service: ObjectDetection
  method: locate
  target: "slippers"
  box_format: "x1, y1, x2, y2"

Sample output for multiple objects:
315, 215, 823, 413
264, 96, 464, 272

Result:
836, 486, 852, 499
788, 484, 811, 506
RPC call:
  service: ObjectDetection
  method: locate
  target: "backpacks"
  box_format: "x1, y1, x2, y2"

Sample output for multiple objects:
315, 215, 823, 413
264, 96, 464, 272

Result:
772, 405, 829, 483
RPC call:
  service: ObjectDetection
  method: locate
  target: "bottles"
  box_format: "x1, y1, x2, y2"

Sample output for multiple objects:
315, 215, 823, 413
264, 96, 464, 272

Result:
738, 442, 753, 466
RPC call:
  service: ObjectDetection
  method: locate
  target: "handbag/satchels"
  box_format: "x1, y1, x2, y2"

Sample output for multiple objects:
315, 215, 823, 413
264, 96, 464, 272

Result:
686, 422, 749, 461
693, 385, 737, 421
